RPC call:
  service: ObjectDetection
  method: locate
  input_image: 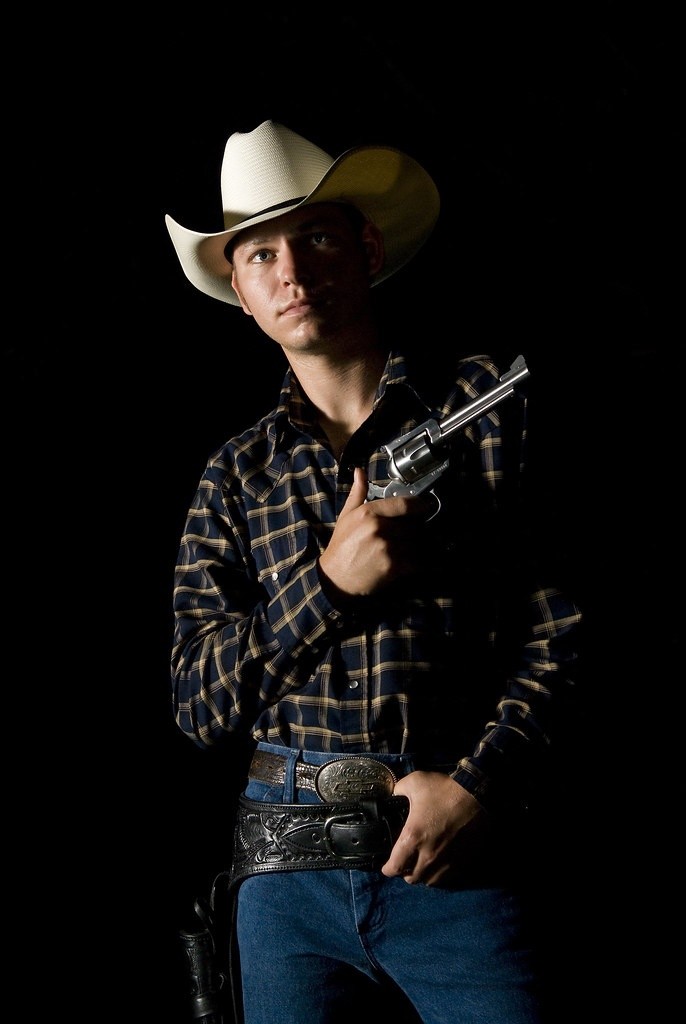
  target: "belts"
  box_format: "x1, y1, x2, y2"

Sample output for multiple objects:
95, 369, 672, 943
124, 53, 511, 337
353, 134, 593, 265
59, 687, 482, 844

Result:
248, 751, 397, 805
226, 792, 409, 890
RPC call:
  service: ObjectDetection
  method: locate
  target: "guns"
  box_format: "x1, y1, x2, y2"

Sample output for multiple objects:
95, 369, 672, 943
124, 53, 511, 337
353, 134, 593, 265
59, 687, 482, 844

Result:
364, 356, 533, 539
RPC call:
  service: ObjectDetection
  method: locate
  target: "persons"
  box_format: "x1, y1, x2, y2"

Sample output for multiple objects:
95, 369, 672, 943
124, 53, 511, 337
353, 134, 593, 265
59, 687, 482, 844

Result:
166, 115, 605, 1023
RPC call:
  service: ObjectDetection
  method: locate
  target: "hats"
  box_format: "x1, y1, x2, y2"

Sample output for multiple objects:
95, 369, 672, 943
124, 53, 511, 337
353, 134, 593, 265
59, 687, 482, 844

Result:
162, 118, 439, 306
